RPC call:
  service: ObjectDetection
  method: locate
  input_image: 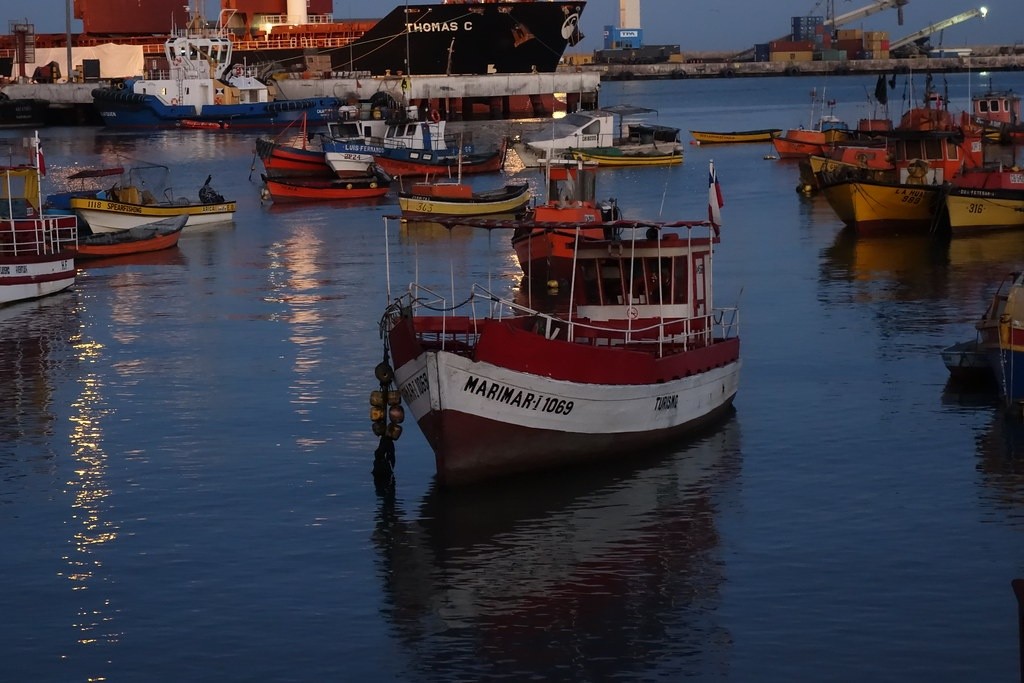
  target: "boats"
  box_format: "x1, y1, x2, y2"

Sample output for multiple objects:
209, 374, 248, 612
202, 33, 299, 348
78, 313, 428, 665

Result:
397, 175, 533, 224
253, 102, 686, 205
369, 155, 740, 503
694, 82, 1024, 420
0, 128, 237, 315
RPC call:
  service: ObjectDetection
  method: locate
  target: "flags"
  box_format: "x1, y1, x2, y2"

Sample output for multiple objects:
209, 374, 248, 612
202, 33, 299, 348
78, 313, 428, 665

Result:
35, 134, 46, 176
708, 165, 724, 237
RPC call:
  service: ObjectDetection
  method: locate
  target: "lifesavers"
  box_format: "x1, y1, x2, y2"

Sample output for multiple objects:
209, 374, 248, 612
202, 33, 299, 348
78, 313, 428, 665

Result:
430, 108, 441, 123
